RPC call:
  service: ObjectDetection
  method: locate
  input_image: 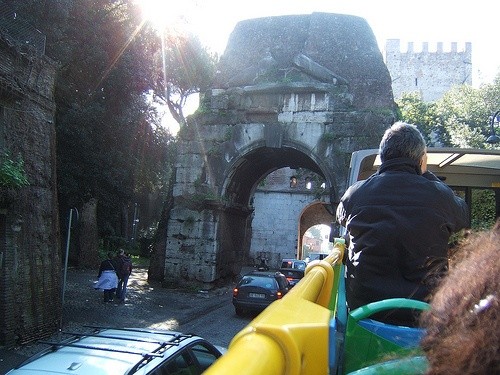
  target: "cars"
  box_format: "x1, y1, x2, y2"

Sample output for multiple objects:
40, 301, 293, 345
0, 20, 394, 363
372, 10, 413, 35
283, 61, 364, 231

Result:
231, 271, 291, 316
278, 253, 330, 289
0, 325, 226, 375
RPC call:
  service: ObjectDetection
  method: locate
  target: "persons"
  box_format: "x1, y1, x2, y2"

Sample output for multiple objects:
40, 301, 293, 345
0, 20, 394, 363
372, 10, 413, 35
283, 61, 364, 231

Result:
99, 253, 117, 303
336, 120, 469, 329
420, 218, 500, 375
114, 249, 132, 302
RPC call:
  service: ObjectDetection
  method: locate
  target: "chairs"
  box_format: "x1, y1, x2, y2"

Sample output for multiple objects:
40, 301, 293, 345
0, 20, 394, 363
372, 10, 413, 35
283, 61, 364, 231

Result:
342, 297, 434, 375
